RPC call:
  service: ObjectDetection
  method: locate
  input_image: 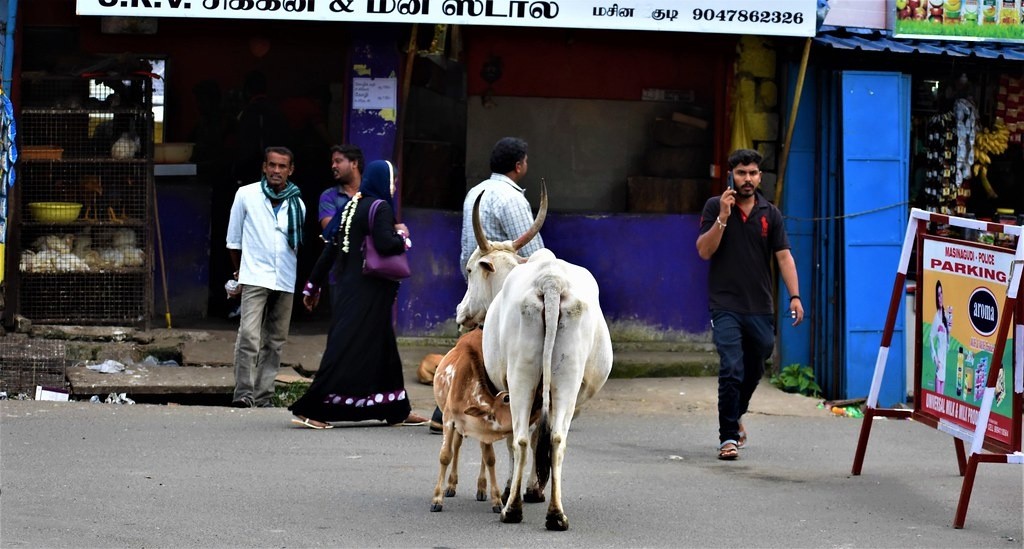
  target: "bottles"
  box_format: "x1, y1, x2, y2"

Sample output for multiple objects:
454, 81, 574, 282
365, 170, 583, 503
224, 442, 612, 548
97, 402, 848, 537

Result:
956, 347, 964, 395
963, 351, 975, 400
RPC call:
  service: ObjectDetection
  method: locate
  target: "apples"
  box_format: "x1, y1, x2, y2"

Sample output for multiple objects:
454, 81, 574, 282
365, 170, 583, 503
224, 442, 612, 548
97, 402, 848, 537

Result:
896, 0, 945, 24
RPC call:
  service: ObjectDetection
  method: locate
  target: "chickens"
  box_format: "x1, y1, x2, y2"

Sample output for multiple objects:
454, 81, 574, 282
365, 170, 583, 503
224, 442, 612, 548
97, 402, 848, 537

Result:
16, 94, 145, 274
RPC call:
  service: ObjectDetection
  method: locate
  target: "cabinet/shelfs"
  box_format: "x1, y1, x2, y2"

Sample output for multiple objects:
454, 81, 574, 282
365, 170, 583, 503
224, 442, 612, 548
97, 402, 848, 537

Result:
12, 72, 154, 334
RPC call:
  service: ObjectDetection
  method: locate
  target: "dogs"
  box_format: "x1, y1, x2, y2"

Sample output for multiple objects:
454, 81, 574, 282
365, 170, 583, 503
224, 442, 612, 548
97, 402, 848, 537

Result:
417, 354, 444, 385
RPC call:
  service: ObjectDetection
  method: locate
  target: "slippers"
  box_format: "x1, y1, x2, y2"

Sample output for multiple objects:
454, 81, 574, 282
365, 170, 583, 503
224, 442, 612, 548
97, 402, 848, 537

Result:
291, 415, 334, 429
392, 412, 431, 426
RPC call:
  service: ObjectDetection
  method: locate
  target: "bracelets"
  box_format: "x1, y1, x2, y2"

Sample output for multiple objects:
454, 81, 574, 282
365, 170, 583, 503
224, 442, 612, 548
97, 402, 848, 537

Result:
788, 295, 801, 303
716, 216, 727, 229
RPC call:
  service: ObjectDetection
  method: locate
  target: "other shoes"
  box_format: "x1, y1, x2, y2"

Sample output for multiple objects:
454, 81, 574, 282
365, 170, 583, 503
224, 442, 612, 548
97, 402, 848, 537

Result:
258, 399, 271, 406
233, 398, 250, 406
430, 425, 443, 433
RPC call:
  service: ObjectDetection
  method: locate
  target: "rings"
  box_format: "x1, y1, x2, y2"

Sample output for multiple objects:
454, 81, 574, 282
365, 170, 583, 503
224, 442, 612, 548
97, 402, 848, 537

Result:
791, 311, 796, 314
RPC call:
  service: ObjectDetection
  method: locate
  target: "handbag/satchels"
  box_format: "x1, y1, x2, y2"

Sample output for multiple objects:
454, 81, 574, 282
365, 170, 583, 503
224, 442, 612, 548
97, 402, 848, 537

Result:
361, 200, 412, 279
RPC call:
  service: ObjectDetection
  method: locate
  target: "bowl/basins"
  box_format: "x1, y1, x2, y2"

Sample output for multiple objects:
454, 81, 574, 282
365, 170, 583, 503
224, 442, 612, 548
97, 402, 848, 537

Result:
154, 142, 196, 164
28, 202, 82, 224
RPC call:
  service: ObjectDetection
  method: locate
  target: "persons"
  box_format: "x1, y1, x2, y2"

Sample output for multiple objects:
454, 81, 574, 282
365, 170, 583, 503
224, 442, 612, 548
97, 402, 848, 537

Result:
696, 149, 805, 457
287, 161, 432, 431
225, 148, 306, 406
429, 138, 544, 433
318, 145, 366, 230
930, 281, 952, 396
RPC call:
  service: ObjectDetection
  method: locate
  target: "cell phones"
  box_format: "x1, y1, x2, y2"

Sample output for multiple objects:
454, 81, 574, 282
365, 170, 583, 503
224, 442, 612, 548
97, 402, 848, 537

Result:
726, 171, 734, 190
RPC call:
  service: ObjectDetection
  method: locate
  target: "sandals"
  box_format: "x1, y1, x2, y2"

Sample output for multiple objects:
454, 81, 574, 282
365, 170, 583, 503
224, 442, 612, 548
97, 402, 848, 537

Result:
717, 439, 738, 458
738, 418, 749, 448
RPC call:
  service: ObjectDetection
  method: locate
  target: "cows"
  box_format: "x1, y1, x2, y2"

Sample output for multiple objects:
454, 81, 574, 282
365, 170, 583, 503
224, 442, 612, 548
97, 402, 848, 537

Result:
429, 178, 613, 531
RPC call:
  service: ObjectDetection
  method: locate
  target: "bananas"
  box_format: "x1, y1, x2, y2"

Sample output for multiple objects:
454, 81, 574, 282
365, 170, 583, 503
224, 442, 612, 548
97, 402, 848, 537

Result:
973, 124, 1011, 176
